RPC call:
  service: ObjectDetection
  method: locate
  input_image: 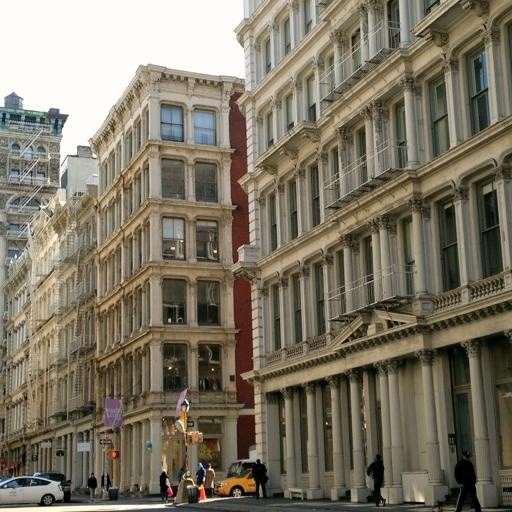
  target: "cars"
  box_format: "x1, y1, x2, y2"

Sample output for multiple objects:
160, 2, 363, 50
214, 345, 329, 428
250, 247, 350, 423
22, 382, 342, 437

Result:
0, 470, 73, 506
214, 457, 259, 498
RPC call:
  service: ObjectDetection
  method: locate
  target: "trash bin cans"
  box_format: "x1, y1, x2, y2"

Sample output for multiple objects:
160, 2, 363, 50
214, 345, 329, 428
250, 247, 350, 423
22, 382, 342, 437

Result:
187, 484, 198, 504
109, 488, 118, 500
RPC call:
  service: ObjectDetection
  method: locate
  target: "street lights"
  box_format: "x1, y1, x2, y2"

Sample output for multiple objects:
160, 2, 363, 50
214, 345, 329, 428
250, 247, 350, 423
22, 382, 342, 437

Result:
181, 399, 189, 472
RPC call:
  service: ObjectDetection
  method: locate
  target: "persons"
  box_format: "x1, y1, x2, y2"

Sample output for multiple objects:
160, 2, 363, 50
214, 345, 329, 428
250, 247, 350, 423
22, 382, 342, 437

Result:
367, 454, 388, 507
195, 461, 215, 498
86, 470, 111, 502
252, 459, 268, 500
158, 472, 172, 503
454, 451, 483, 512
